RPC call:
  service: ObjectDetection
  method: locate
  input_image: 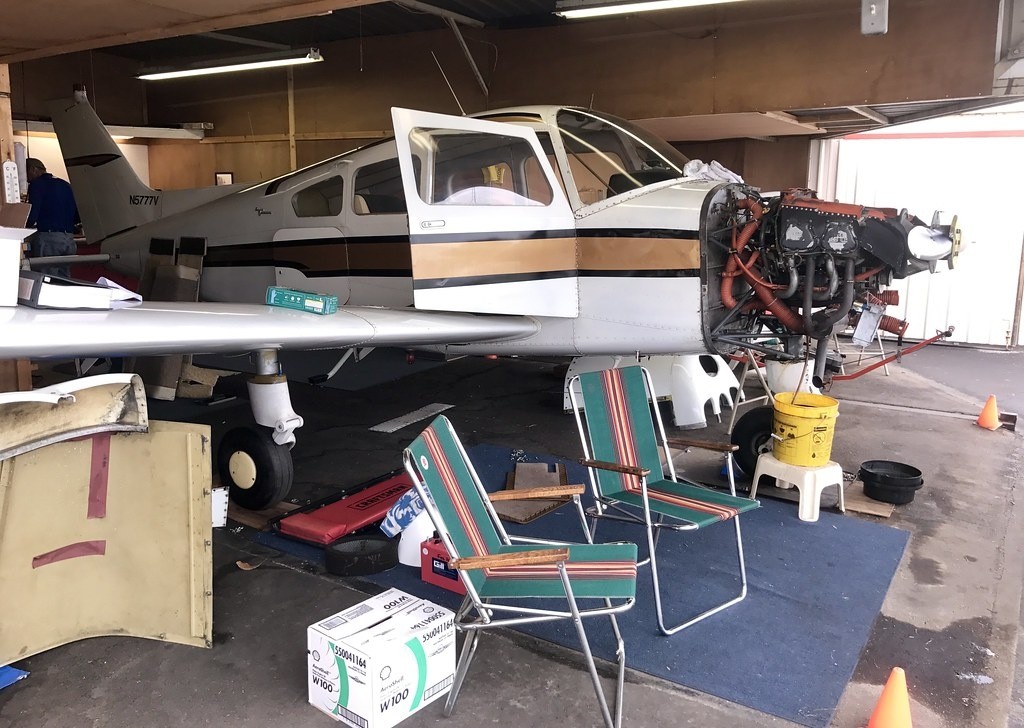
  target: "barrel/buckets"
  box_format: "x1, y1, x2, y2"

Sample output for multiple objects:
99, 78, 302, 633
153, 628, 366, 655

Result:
773, 392, 840, 467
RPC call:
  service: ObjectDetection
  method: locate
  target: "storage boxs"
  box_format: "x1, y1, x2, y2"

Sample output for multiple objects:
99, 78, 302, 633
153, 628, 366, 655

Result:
265, 285, 339, 316
0, 201, 39, 308
306, 587, 456, 728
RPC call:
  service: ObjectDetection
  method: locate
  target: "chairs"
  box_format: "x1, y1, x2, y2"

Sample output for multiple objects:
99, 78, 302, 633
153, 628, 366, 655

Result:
403, 414, 639, 728
567, 365, 761, 636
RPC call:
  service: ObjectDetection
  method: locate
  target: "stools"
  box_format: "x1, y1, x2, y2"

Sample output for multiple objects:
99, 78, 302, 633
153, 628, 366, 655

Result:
749, 450, 846, 522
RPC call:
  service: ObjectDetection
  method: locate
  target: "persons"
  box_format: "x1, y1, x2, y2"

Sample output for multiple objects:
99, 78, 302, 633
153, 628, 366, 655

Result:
25, 158, 80, 279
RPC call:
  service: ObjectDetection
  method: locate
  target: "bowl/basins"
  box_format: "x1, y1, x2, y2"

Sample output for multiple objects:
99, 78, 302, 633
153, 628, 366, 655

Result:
857, 460, 924, 506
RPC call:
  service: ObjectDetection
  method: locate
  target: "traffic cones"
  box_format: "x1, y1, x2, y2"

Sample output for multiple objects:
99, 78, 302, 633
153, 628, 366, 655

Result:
860, 666, 911, 728
972, 394, 1004, 431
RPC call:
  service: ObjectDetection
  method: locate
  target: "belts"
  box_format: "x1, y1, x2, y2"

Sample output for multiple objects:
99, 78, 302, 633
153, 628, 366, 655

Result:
40, 230, 72, 233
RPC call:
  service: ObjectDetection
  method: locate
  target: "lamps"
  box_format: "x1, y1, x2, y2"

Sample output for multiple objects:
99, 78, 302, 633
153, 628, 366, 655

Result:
550, 0, 741, 19
130, 44, 326, 80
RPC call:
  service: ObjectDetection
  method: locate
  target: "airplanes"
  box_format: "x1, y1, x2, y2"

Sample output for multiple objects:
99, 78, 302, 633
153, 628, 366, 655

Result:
1, 86, 962, 510
